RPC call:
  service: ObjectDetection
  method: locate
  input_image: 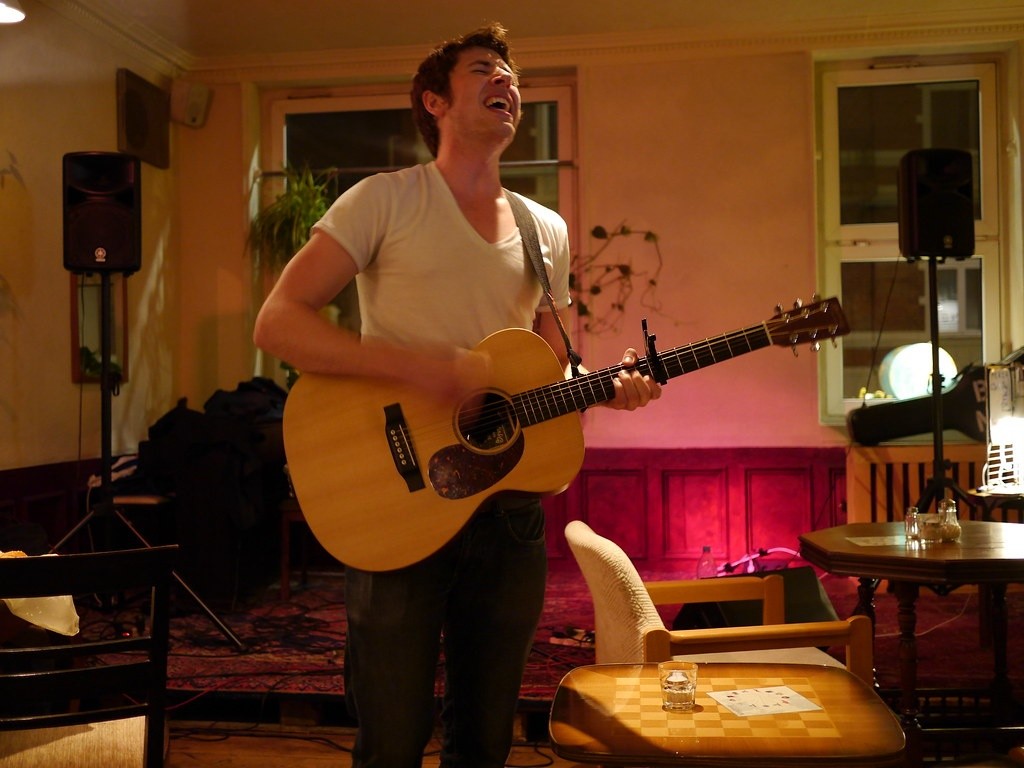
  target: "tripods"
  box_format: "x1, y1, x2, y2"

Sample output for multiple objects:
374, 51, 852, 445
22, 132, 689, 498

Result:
51, 272, 248, 654
852, 259, 979, 616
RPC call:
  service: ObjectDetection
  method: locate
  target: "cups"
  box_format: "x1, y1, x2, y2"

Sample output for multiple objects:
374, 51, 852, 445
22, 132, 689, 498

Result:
917, 514, 939, 544
658, 660, 698, 709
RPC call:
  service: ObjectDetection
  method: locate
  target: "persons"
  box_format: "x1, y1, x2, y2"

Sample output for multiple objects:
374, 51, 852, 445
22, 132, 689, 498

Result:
252, 25, 662, 767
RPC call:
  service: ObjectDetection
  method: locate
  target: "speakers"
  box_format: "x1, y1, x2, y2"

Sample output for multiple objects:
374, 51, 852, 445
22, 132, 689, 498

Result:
62, 151, 142, 278
184, 83, 211, 126
896, 147, 975, 264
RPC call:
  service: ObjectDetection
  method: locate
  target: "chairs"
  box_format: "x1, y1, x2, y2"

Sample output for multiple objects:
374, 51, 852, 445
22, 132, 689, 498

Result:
564, 520, 875, 689
0, 542, 180, 768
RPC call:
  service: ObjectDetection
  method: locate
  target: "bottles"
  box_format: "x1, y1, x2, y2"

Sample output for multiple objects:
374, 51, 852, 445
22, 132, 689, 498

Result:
937, 498, 961, 543
905, 507, 919, 538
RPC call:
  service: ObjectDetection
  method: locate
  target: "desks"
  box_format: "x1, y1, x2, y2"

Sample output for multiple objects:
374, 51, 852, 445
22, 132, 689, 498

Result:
798, 520, 1024, 768
548, 663, 906, 768
279, 501, 310, 604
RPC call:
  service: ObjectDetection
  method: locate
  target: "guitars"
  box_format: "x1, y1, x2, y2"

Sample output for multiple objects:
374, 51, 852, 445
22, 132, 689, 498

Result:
279, 288, 859, 578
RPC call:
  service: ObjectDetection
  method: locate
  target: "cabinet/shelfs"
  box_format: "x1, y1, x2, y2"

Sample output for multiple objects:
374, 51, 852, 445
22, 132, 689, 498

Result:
812, 60, 1007, 428
